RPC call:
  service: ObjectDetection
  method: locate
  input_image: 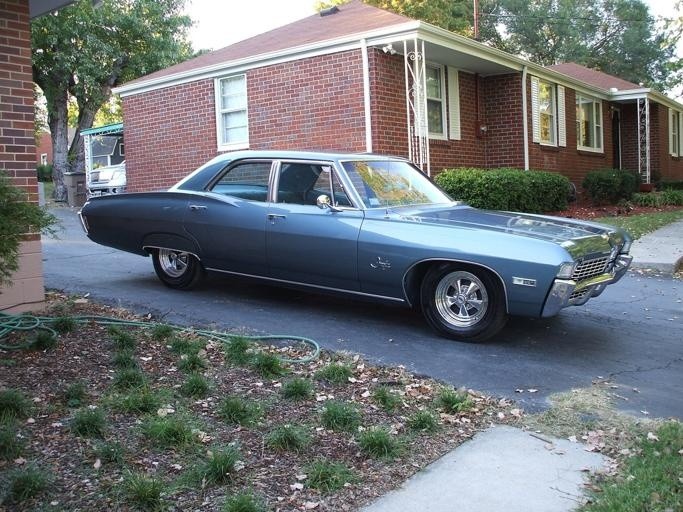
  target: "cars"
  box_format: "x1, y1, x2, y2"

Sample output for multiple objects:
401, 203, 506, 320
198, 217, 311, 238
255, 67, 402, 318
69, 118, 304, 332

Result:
77, 149, 634, 343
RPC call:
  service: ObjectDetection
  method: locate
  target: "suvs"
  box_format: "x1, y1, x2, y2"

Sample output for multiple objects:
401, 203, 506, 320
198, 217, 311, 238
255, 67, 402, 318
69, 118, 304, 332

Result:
85, 160, 125, 198
